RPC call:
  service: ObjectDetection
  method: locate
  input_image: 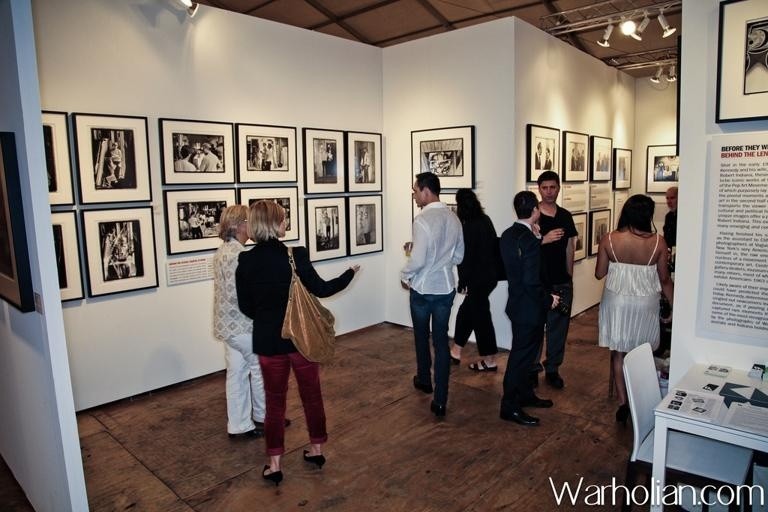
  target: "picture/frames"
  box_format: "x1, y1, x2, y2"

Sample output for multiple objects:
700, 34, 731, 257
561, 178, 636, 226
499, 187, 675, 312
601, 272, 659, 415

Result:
411, 125, 474, 189
0, 131, 35, 314
525, 124, 677, 262
714, 1, 767, 122
412, 193, 457, 223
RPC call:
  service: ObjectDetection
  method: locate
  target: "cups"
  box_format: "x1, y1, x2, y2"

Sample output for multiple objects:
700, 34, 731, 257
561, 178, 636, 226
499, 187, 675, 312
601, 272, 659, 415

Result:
559, 228, 567, 240
552, 291, 562, 296
405, 243, 415, 259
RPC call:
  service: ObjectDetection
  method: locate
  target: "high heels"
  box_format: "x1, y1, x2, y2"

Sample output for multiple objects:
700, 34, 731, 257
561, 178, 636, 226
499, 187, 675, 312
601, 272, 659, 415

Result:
300, 448, 326, 471
614, 404, 631, 426
261, 464, 282, 485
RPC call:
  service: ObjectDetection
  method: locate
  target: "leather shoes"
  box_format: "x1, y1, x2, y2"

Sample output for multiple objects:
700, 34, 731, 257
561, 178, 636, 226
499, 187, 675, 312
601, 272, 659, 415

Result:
544, 370, 564, 389
254, 417, 291, 428
521, 394, 554, 409
432, 399, 446, 418
530, 373, 539, 389
412, 374, 435, 395
227, 427, 265, 441
500, 406, 539, 426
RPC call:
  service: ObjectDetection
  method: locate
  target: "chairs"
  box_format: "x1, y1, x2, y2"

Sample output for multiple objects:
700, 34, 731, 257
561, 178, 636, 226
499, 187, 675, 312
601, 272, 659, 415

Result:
622, 342, 755, 512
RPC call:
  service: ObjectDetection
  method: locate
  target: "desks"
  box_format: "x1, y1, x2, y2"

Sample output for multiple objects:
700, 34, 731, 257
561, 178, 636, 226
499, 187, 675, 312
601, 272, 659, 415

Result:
650, 363, 768, 512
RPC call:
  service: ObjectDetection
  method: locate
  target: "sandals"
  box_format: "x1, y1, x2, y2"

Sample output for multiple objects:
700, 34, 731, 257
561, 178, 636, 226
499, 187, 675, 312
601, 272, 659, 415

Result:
468, 361, 498, 372
449, 353, 461, 366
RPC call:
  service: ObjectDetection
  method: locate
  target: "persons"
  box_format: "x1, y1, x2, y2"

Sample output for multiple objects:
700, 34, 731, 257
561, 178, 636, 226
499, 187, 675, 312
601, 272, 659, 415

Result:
236, 199, 360, 486
500, 190, 561, 424
450, 188, 501, 372
597, 152, 608, 171
535, 169, 579, 389
571, 148, 585, 170
400, 172, 467, 417
321, 145, 334, 177
110, 143, 122, 180
323, 210, 331, 241
662, 187, 678, 250
212, 204, 291, 440
261, 141, 273, 170
362, 212, 371, 244
112, 237, 131, 278
594, 194, 673, 427
175, 143, 222, 171
188, 210, 215, 239
362, 147, 372, 183
535, 142, 552, 170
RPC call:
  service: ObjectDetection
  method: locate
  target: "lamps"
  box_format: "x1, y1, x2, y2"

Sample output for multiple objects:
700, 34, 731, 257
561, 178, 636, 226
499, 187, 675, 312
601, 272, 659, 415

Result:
597, 8, 676, 83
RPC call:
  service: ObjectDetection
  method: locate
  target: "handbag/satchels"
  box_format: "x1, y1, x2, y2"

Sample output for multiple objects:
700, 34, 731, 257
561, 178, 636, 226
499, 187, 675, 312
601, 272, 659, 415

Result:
282, 245, 337, 364
